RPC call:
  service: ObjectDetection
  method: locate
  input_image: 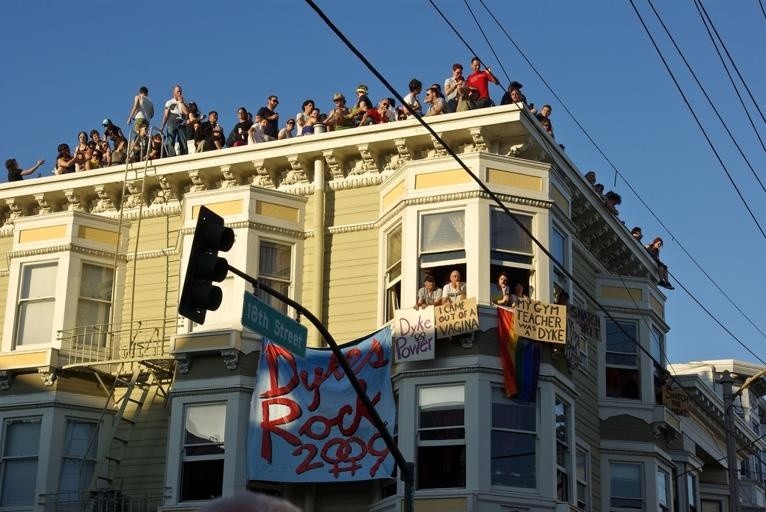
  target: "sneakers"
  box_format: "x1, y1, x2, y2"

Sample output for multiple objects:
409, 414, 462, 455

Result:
658, 281, 668, 288
665, 282, 675, 289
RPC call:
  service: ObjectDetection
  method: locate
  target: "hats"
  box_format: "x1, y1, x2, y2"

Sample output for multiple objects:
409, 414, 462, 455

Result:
333, 92, 345, 102
356, 85, 368, 95
508, 81, 524, 92
101, 118, 112, 125
425, 275, 435, 281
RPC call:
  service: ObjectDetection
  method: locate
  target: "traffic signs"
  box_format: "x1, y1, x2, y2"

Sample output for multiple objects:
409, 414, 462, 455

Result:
240, 290, 308, 357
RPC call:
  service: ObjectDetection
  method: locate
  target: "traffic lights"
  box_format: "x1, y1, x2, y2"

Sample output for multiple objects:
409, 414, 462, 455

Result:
177, 206, 235, 325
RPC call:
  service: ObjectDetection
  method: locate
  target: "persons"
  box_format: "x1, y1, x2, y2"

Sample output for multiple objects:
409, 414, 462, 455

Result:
54, 118, 128, 175
583, 168, 675, 292
128, 84, 225, 164
444, 57, 498, 113
348, 78, 449, 128
507, 282, 530, 305
440, 270, 466, 305
654, 369, 670, 394
500, 81, 565, 151
227, 93, 353, 149
488, 273, 510, 308
6, 159, 46, 181
412, 274, 442, 311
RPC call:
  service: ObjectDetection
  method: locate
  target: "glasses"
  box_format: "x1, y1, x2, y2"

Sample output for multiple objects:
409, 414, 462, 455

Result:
273, 99, 279, 104
288, 122, 295, 125
65, 128, 159, 158
383, 103, 389, 107
